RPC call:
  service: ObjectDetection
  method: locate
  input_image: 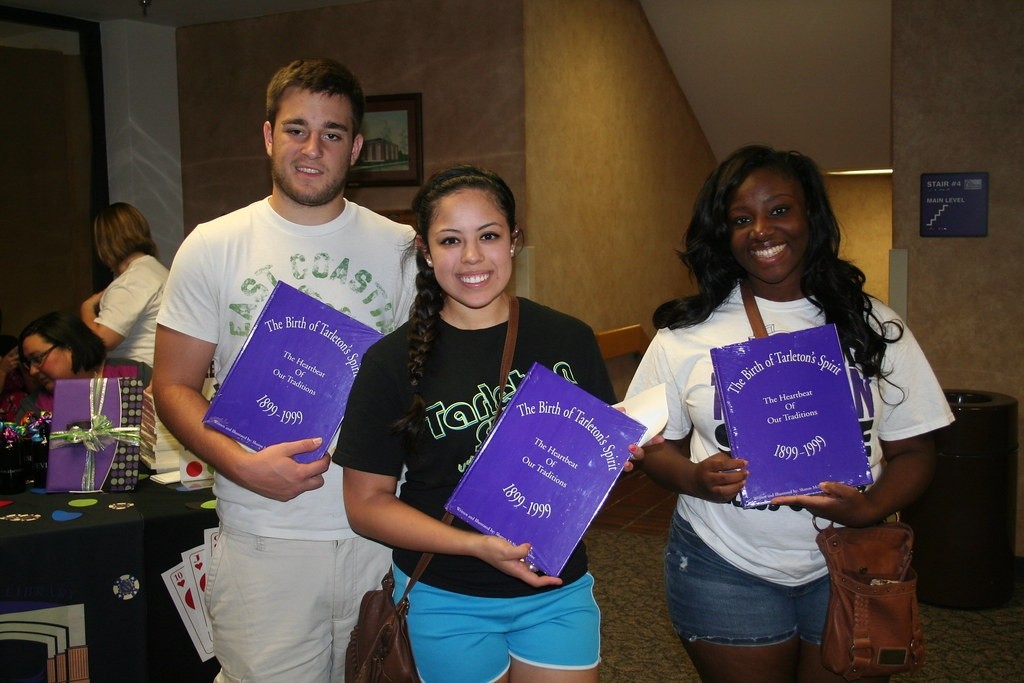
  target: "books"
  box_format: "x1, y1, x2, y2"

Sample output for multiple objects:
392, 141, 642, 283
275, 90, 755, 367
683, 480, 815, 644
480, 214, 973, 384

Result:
446, 362, 647, 576
201, 280, 385, 463
710, 323, 873, 509
140, 379, 216, 469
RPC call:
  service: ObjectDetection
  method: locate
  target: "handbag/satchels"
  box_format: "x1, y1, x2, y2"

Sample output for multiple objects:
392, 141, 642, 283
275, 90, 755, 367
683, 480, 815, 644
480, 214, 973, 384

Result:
346, 591, 419, 683
816, 521, 926, 681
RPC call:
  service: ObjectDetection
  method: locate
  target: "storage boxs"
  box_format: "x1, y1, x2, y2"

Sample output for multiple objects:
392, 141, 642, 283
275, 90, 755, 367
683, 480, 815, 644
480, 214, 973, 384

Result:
48, 377, 144, 491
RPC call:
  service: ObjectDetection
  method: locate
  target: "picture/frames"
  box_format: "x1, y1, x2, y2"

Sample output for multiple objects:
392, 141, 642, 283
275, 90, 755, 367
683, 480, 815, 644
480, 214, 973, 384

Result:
343, 92, 423, 187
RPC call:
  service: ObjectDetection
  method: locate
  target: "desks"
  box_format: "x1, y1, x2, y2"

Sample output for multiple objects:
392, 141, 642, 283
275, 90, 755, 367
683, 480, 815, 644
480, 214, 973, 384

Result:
0, 486, 219, 683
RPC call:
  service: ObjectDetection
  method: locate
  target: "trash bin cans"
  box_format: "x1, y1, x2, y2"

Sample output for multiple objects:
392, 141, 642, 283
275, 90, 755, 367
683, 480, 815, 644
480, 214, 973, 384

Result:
899, 389, 1018, 610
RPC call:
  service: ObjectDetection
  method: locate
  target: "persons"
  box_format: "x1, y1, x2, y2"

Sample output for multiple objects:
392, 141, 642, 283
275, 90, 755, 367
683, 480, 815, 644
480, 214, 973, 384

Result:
623, 145, 956, 683
151, 60, 432, 683
331, 165, 665, 683
0, 312, 152, 486
81, 202, 169, 368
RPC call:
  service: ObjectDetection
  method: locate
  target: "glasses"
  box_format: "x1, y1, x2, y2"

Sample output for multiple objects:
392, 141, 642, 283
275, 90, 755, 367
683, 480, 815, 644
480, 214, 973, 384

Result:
22, 343, 59, 369
